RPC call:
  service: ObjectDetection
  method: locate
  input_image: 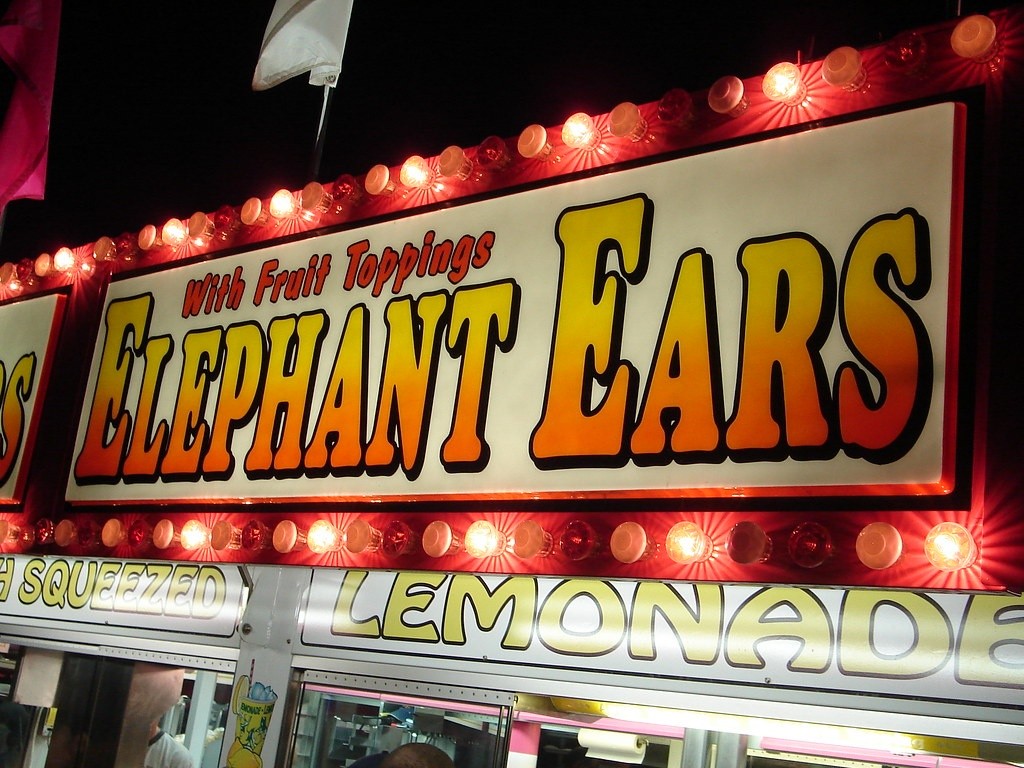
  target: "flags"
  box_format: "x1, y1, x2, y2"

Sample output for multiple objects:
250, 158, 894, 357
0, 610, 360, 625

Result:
1, 0, 60, 219
252, 0, 354, 91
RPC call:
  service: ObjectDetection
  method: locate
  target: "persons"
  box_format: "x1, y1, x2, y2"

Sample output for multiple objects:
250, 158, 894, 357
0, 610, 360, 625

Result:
144, 714, 194, 768
